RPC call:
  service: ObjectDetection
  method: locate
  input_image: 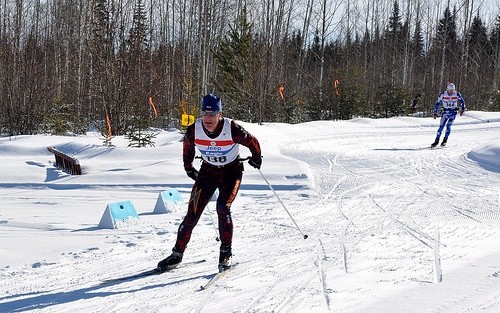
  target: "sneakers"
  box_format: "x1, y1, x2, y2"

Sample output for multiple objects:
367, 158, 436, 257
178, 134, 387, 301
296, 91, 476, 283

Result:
157, 246, 185, 271
218, 243, 233, 273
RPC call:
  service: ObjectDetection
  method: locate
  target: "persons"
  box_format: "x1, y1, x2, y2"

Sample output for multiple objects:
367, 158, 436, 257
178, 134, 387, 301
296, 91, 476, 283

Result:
431, 83, 465, 147
158, 94, 262, 269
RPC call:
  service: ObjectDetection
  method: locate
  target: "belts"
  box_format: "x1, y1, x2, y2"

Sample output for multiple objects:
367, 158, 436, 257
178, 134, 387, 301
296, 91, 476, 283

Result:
204, 157, 239, 168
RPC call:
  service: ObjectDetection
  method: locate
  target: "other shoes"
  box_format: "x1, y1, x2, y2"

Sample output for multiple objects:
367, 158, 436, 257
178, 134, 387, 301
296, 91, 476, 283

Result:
441, 137, 448, 146
433, 138, 439, 146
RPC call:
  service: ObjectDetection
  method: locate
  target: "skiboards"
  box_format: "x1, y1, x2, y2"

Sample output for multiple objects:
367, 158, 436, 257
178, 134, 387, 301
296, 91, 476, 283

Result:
98, 259, 240, 291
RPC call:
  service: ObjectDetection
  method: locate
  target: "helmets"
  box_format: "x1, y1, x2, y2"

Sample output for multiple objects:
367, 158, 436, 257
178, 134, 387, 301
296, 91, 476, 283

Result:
447, 83, 456, 91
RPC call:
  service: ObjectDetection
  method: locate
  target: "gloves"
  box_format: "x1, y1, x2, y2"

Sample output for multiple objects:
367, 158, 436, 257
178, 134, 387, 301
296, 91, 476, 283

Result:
433, 112, 439, 119
247, 156, 262, 170
460, 110, 465, 116
184, 166, 199, 181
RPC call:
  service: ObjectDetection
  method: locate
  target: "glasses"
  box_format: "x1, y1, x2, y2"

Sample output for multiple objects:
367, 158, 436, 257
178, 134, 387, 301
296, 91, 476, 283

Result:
200, 110, 221, 117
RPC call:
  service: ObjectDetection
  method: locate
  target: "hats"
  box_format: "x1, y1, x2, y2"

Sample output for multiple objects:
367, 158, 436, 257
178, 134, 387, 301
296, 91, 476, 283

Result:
201, 94, 222, 112
